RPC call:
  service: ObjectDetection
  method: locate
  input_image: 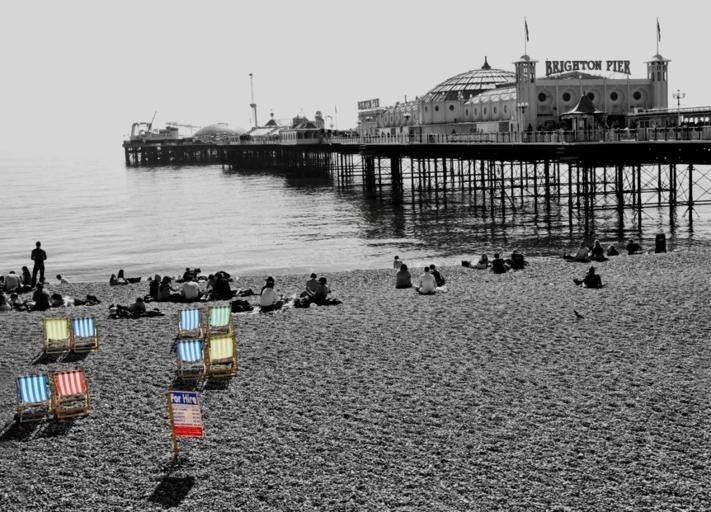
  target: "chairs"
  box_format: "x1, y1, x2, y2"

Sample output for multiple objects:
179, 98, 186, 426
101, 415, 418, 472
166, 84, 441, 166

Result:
173, 306, 239, 382
14, 314, 98, 423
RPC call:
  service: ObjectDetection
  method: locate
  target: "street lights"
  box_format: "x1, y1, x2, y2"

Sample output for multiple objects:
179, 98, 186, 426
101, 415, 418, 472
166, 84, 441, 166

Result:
517, 102, 529, 130
672, 88, 686, 127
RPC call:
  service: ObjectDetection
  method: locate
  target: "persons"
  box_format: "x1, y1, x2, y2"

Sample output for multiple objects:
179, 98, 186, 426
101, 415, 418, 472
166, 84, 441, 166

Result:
588, 240, 608, 262
260, 276, 283, 312
492, 253, 508, 273
31, 241, 47, 283
626, 240, 640, 255
0, 266, 54, 310
511, 251, 524, 270
584, 267, 603, 289
51, 293, 84, 307
393, 256, 445, 295
56, 275, 72, 288
607, 246, 619, 256
563, 242, 590, 261
110, 269, 128, 286
479, 254, 488, 268
117, 297, 146, 314
296, 274, 328, 309
149, 267, 233, 303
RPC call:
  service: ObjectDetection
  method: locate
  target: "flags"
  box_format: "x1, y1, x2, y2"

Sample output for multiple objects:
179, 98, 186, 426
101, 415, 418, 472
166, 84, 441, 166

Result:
525, 18, 529, 42
656, 19, 661, 42
